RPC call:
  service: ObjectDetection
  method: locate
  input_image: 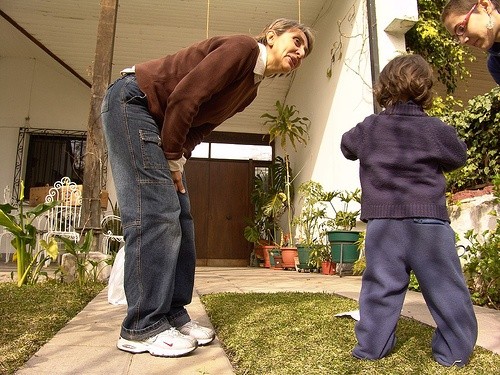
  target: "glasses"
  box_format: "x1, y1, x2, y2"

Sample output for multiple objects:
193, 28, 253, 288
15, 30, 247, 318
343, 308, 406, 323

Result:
452, 3, 477, 43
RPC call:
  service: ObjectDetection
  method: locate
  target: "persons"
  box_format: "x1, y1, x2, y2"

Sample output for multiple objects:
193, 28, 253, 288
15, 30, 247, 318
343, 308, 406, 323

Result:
340, 54, 479, 367
441, 0, 500, 87
99, 17, 314, 358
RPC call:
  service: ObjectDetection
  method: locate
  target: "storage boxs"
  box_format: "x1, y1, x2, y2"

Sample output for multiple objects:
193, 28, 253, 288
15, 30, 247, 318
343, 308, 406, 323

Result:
29, 184, 108, 207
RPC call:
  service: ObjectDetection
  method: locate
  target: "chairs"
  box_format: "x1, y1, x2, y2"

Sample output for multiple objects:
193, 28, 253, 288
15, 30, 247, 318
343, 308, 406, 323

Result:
38, 177, 82, 269
0, 185, 19, 264
100, 215, 125, 255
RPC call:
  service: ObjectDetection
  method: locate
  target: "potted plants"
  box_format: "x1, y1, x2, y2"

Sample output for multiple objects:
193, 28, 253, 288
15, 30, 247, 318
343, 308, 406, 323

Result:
243, 100, 362, 276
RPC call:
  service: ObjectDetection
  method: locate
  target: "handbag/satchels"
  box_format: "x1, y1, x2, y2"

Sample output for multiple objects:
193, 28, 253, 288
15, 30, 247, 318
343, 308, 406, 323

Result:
108, 247, 129, 306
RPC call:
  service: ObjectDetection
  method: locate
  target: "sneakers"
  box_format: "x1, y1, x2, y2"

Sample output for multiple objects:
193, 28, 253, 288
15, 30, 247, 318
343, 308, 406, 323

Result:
117, 327, 198, 357
176, 320, 215, 346
